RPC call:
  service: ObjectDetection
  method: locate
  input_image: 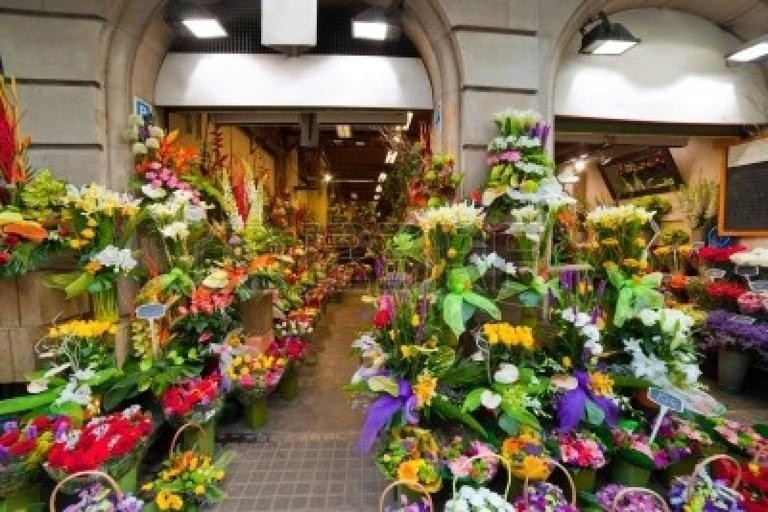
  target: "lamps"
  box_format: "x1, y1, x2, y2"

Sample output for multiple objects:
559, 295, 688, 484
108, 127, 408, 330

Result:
578, 12, 641, 57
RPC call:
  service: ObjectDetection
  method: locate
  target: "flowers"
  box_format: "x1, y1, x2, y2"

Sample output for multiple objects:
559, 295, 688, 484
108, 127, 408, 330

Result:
1, 72, 225, 510
225, 155, 353, 401
352, 106, 768, 509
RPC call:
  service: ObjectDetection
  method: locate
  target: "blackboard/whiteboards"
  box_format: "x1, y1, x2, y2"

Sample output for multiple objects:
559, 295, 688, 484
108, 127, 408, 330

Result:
718, 131, 768, 237
